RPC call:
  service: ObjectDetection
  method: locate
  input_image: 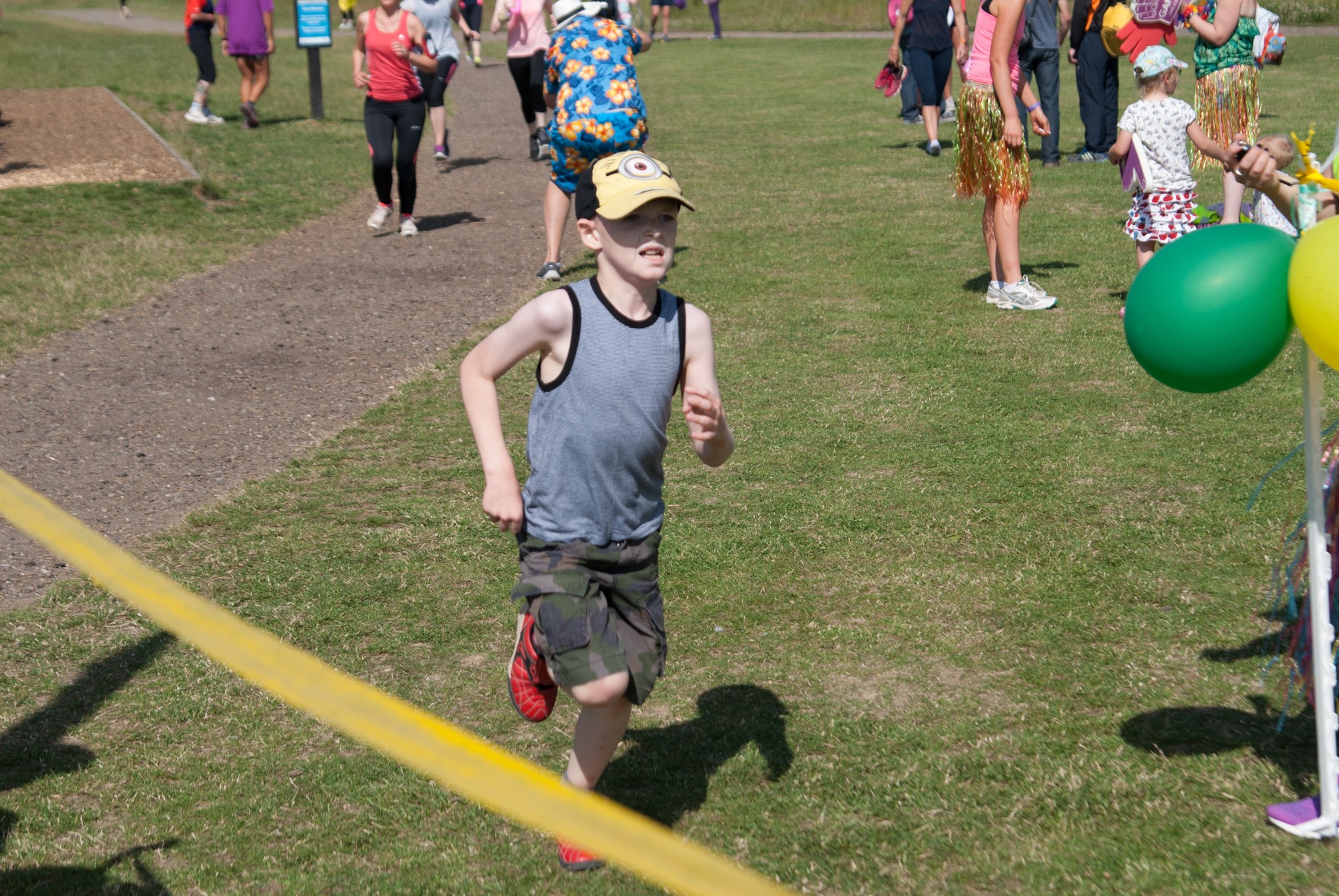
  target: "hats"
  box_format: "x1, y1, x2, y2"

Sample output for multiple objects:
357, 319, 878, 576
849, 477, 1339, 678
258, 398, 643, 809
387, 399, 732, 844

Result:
545, 0, 601, 34
574, 150, 695, 222
1133, 45, 1189, 79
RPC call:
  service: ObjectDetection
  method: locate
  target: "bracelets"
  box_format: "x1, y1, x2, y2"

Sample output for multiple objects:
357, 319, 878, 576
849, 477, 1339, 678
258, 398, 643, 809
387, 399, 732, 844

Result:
959, 38, 967, 45
222, 37, 228, 41
1183, 3, 1196, 18
1186, 11, 1195, 22
1027, 101, 1041, 112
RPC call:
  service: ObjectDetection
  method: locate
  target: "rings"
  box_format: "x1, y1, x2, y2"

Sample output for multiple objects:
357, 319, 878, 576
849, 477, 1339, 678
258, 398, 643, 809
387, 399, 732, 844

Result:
1235, 166, 1247, 177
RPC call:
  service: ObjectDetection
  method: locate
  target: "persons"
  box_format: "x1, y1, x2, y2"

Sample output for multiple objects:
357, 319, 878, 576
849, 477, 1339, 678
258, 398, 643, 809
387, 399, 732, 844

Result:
648, 0, 674, 43
1106, 45, 1232, 319
214, 0, 275, 129
399, 0, 481, 161
950, 0, 1058, 311
183, 0, 223, 125
490, 0, 558, 161
353, 0, 438, 235
458, 150, 736, 870
704, 0, 723, 40
887, 0, 1124, 168
534, 0, 651, 282
1224, 133, 1339, 838
337, 0, 686, 69
1177, 0, 1262, 225
118, 0, 131, 18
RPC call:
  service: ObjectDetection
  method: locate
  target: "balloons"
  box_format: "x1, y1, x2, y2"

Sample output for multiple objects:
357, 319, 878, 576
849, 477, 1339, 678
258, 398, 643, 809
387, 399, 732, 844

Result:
1286, 215, 1339, 374
1124, 222, 1297, 393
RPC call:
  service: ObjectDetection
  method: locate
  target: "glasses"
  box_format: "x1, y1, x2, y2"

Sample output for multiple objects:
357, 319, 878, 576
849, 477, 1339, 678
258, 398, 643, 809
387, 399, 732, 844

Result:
1176, 68, 1182, 75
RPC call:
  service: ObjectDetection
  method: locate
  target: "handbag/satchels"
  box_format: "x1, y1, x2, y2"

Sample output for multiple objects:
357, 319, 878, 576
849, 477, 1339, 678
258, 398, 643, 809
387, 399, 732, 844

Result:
1255, 22, 1287, 66
1018, 23, 1033, 48
1100, 2, 1134, 57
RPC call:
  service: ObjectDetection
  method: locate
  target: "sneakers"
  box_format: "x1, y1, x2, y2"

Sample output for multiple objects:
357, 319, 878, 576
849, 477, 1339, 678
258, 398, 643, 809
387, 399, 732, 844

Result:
1263, 794, 1323, 838
366, 203, 418, 237
997, 274, 1058, 310
1067, 146, 1109, 163
986, 280, 1002, 304
536, 127, 550, 146
529, 133, 543, 161
433, 143, 449, 161
557, 834, 606, 870
507, 602, 558, 723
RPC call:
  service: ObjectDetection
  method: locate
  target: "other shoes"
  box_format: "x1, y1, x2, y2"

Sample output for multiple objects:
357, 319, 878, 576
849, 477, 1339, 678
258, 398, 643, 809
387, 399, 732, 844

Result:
1044, 159, 1061, 167
240, 102, 258, 128
184, 107, 223, 125
463, 48, 482, 65
1120, 307, 1125, 318
536, 262, 561, 281
875, 61, 958, 156
